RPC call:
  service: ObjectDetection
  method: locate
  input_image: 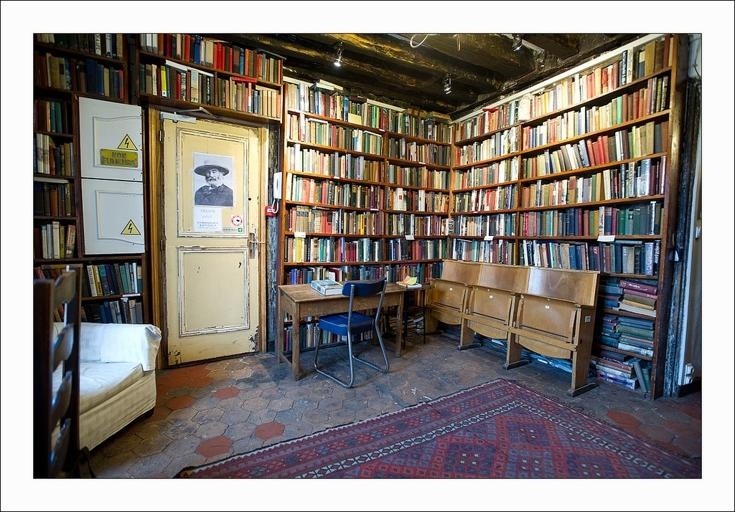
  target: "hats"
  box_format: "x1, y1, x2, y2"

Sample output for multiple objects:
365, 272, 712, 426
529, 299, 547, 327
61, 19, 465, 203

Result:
194, 160, 229, 176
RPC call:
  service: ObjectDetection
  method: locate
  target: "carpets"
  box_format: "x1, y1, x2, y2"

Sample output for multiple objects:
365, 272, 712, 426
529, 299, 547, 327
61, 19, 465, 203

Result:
172, 380, 702, 479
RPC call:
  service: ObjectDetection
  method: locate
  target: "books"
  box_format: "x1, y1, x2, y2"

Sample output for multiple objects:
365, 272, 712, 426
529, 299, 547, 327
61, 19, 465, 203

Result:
32, 33, 281, 121
282, 78, 447, 297
34, 121, 78, 326
77, 260, 146, 326
452, 37, 674, 275
590, 275, 658, 398
282, 313, 425, 358
482, 337, 574, 373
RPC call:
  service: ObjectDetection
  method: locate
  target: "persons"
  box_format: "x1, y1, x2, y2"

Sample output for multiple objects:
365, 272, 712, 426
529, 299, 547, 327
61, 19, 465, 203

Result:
193, 157, 234, 208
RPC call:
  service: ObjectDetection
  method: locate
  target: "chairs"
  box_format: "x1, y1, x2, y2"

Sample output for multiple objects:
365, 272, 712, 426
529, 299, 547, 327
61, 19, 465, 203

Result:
53, 320, 160, 453
